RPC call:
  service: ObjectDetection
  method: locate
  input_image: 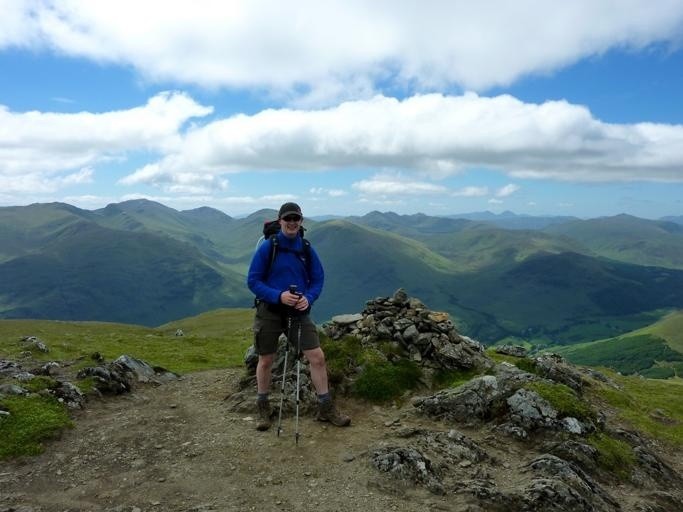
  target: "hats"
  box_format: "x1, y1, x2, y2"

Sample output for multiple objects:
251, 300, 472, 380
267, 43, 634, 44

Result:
279, 202, 302, 218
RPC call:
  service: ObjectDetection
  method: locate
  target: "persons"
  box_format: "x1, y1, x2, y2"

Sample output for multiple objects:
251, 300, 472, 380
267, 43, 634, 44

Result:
246, 202, 351, 430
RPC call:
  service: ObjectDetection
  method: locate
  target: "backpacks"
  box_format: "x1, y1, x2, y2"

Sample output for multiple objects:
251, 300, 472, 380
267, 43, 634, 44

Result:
255, 219, 310, 302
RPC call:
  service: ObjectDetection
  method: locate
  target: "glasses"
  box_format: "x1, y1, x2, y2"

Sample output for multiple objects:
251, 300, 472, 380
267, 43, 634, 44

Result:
282, 214, 300, 222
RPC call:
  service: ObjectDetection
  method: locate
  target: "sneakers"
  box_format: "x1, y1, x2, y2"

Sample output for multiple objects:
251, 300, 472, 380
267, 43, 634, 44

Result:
318, 400, 350, 426
255, 398, 274, 430
